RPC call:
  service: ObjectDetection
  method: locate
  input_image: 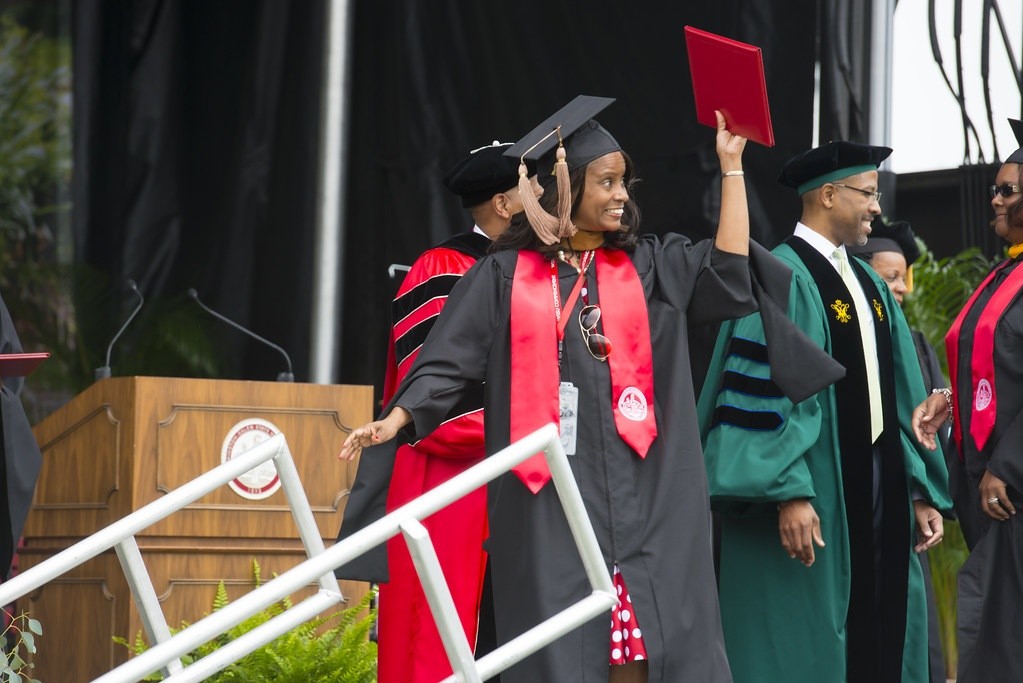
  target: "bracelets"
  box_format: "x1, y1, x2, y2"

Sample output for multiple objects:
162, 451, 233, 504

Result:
932, 388, 954, 422
722, 170, 744, 177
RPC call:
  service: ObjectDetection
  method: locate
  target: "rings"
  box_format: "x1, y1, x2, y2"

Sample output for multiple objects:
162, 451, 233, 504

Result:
987, 497, 998, 504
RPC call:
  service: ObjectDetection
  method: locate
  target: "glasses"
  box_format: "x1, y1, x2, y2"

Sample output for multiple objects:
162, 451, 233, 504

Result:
832, 180, 883, 206
578, 306, 612, 363
989, 183, 1023, 200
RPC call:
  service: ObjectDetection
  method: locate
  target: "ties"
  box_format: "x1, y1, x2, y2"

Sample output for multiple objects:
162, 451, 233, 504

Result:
834, 246, 849, 288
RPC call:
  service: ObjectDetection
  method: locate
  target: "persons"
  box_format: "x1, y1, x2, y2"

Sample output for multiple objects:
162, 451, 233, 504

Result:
847, 213, 957, 683
334, 141, 546, 683
698, 141, 957, 683
336, 94, 846, 683
912, 117, 1023, 683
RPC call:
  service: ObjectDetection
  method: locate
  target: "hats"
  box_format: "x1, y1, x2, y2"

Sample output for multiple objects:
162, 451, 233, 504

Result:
443, 142, 543, 209
778, 140, 893, 199
1004, 118, 1023, 167
502, 93, 622, 244
848, 216, 920, 293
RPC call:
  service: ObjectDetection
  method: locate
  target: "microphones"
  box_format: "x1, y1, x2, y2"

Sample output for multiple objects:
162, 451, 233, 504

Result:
95, 278, 144, 382
186, 288, 294, 383
